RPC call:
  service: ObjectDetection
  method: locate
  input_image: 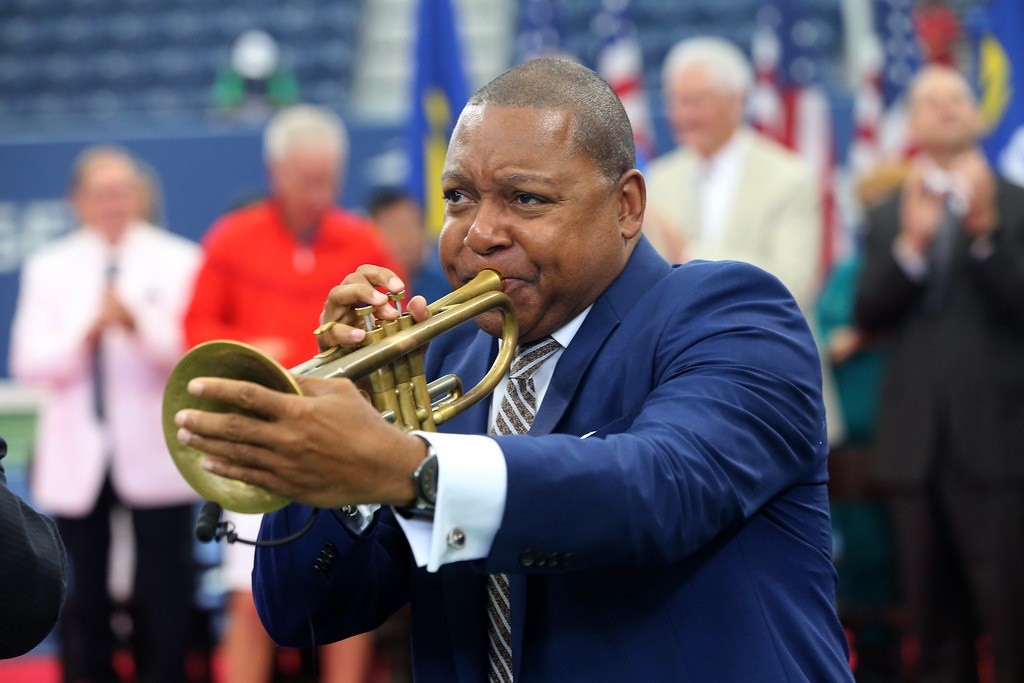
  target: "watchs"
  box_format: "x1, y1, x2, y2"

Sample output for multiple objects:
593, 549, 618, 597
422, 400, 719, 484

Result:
396, 433, 438, 522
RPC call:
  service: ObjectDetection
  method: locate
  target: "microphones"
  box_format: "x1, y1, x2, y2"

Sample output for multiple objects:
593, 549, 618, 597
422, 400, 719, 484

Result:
196, 501, 327, 548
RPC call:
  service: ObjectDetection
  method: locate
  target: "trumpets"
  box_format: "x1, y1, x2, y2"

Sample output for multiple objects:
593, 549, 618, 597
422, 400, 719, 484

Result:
160, 267, 523, 516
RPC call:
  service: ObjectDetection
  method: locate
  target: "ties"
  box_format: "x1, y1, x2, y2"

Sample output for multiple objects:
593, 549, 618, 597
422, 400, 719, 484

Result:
485, 338, 562, 683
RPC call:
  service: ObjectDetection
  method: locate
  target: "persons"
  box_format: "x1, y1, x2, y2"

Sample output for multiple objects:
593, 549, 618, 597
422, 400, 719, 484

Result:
361, 183, 456, 306
6, 141, 208, 682
852, 65, 1024, 682
172, 58, 857, 683
0, 436, 71, 659
180, 103, 410, 683
813, 251, 907, 681
636, 34, 849, 449
